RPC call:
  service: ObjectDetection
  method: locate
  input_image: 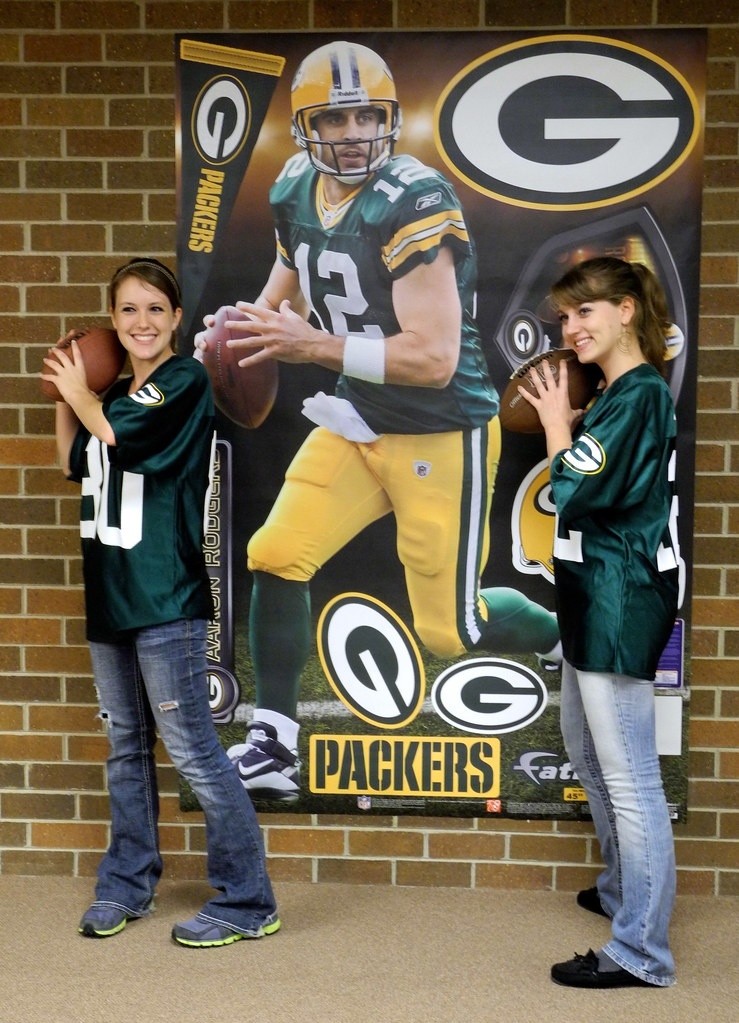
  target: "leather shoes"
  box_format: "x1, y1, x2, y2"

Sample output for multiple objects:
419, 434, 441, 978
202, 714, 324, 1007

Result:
577, 886, 615, 921
550, 946, 669, 989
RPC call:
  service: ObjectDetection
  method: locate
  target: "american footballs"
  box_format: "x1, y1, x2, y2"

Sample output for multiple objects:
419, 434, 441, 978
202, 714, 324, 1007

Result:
203, 306, 280, 428
41, 326, 128, 401
498, 349, 591, 435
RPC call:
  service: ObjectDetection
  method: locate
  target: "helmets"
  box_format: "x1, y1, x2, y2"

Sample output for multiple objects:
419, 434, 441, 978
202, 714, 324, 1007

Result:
290, 40, 399, 162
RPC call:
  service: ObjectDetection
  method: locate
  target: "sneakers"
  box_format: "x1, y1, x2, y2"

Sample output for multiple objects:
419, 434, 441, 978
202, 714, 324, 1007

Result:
77, 887, 158, 939
226, 720, 302, 805
171, 912, 280, 949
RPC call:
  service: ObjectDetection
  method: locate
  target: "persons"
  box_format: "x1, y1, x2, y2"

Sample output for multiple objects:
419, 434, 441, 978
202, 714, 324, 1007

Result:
517, 254, 682, 989
188, 38, 566, 797
38, 256, 281, 948
